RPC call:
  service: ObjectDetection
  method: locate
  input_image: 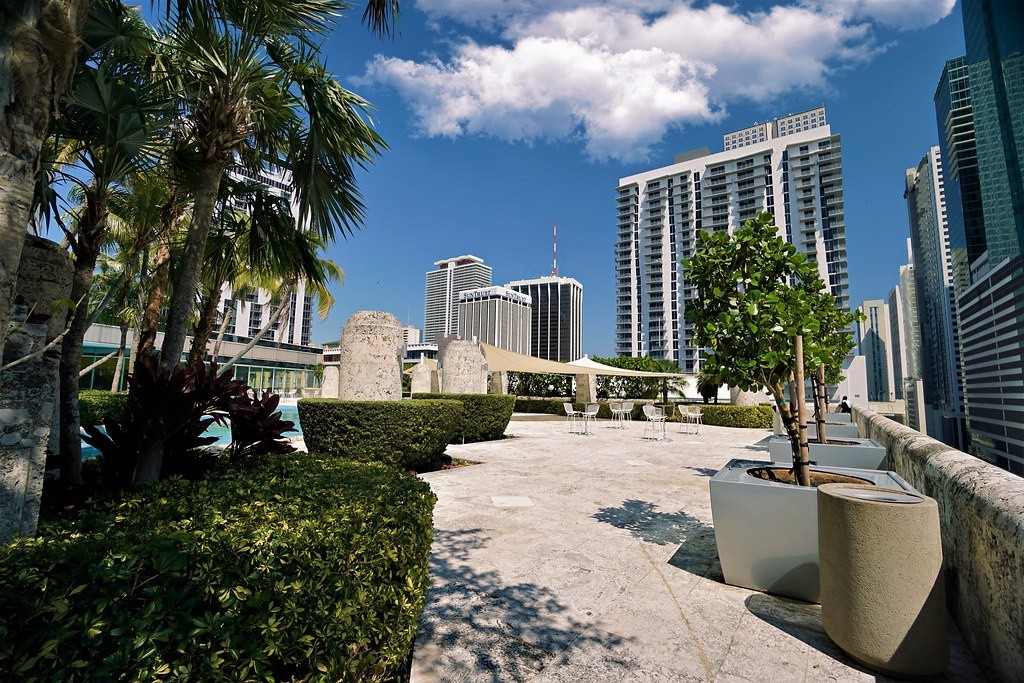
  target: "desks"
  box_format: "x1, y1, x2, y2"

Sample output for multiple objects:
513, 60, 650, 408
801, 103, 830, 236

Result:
693, 413, 703, 435
616, 410, 624, 428
580, 412, 591, 436
654, 417, 665, 441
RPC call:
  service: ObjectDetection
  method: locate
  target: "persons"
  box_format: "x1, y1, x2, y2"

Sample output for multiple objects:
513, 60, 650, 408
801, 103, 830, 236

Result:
838, 395, 852, 413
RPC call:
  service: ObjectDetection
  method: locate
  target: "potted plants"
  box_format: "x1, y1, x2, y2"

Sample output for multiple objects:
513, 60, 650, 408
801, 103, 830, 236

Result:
681, 213, 920, 604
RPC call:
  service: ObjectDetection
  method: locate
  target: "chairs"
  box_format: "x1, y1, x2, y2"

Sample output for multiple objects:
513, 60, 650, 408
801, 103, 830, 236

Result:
609, 402, 634, 430
564, 403, 582, 435
678, 405, 704, 436
642, 406, 668, 441
579, 404, 600, 435
